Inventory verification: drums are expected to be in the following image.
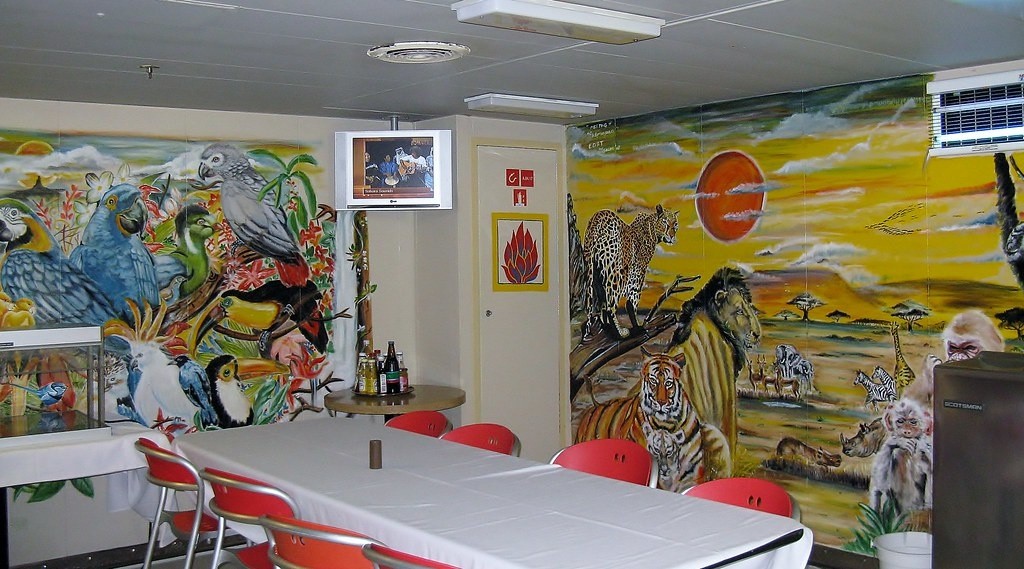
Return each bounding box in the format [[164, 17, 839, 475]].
[[385, 176, 399, 188]]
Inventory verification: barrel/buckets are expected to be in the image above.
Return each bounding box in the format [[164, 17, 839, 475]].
[[873, 531, 932, 569]]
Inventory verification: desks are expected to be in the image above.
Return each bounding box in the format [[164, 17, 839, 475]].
[[323, 384, 466, 423], [1, 409, 166, 569], [931, 351, 1024, 568]]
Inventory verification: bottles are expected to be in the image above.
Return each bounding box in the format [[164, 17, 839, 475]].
[[365, 360, 378, 395], [383, 340, 400, 393], [358, 339, 374, 362], [373, 350, 383, 359], [368, 353, 378, 361], [395, 352, 405, 369], [377, 357, 388, 394], [358, 359, 368, 394]]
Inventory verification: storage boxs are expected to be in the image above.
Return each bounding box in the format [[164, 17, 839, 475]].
[[0, 323, 112, 448]]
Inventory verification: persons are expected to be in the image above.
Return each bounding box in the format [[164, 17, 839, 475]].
[[366, 146, 433, 191]]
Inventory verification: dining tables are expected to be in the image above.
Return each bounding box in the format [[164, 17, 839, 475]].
[[174, 417, 814, 569]]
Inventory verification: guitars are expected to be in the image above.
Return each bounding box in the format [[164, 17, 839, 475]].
[[399, 162, 427, 176]]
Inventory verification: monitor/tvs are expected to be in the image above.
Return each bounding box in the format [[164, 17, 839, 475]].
[[335, 129, 453, 209]]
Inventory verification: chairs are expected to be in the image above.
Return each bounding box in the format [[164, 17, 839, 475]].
[[131, 410, 803, 569]]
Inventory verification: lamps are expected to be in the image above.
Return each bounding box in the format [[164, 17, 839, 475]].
[[450, 0, 666, 46], [463, 93, 600, 119]]
